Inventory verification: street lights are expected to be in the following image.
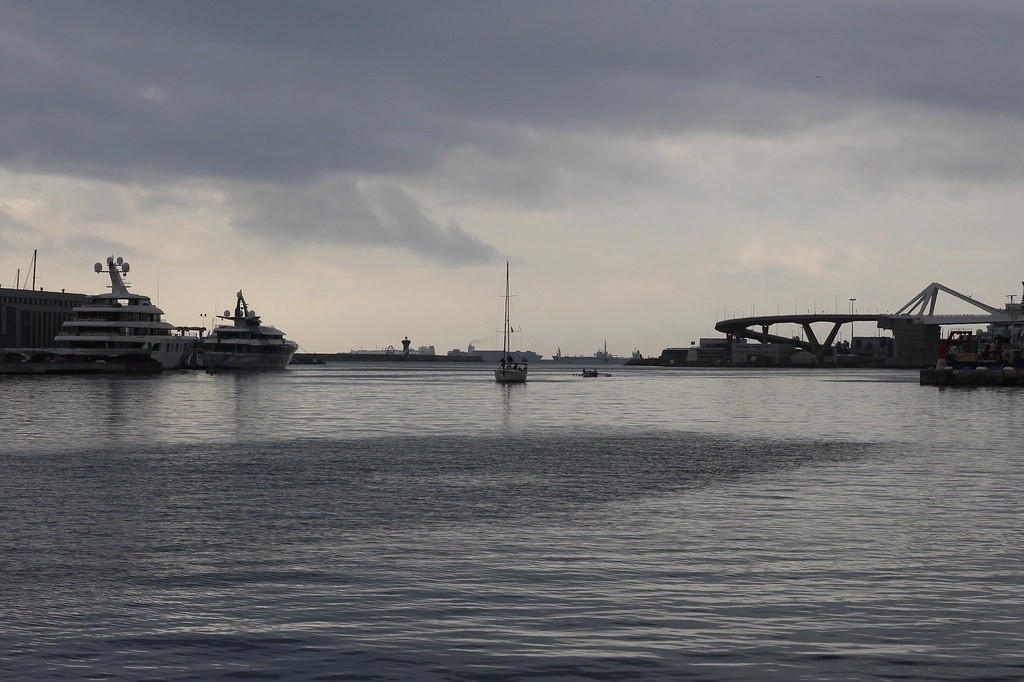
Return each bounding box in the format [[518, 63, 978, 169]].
[[849, 297, 856, 339]]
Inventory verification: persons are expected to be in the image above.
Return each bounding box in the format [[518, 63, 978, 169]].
[[583, 367, 597, 373], [937, 342, 947, 366], [500, 357, 522, 371]]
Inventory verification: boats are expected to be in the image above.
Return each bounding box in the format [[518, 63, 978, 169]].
[[581, 367, 611, 377], [48, 253, 299, 371]]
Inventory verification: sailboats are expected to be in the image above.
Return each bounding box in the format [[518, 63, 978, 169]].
[[494, 259, 527, 383]]
